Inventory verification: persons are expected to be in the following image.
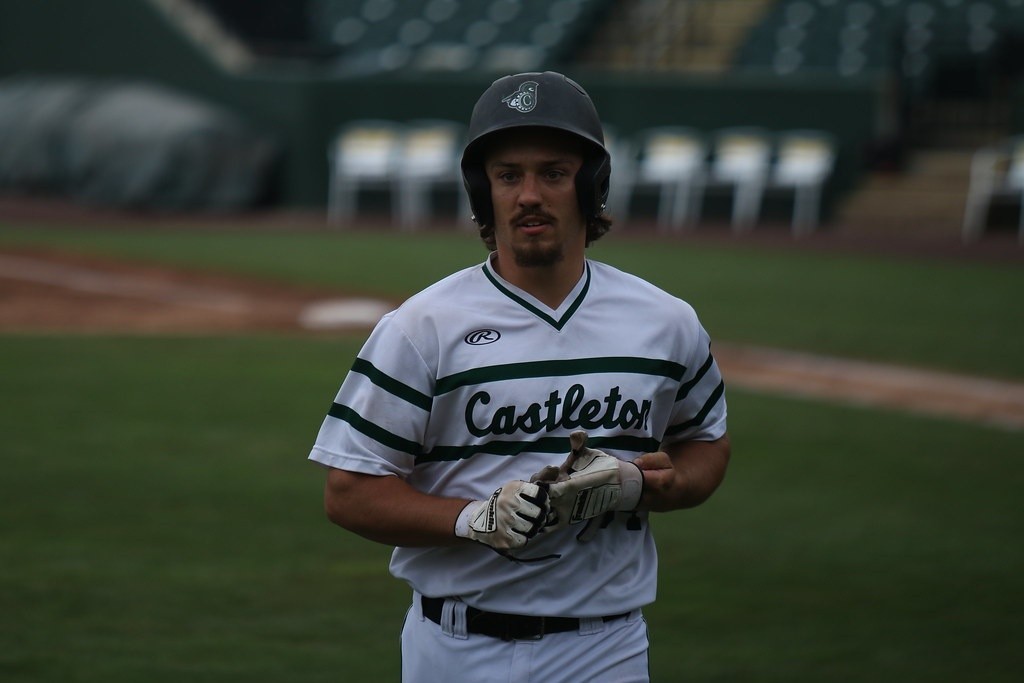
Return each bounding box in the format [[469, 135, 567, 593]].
[[306, 72, 728, 683]]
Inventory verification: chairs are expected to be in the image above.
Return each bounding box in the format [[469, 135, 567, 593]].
[[325, 126, 836, 236]]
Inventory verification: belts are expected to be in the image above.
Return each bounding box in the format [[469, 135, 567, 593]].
[[421, 595, 630, 641]]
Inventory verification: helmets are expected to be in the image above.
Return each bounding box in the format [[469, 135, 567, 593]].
[[460, 71, 610, 226]]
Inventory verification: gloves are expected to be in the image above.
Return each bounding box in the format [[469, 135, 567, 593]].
[[535, 431, 644, 542], [454, 479, 549, 549]]
